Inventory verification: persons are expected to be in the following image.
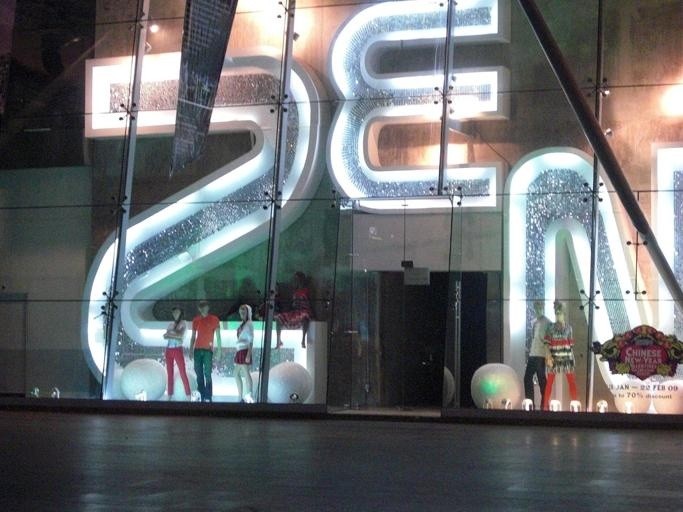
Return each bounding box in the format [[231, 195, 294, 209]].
[[542, 299, 578, 411], [189, 300, 221, 403], [232, 304, 255, 403], [524, 300, 551, 409], [275, 271, 311, 350], [162, 306, 191, 400]]
